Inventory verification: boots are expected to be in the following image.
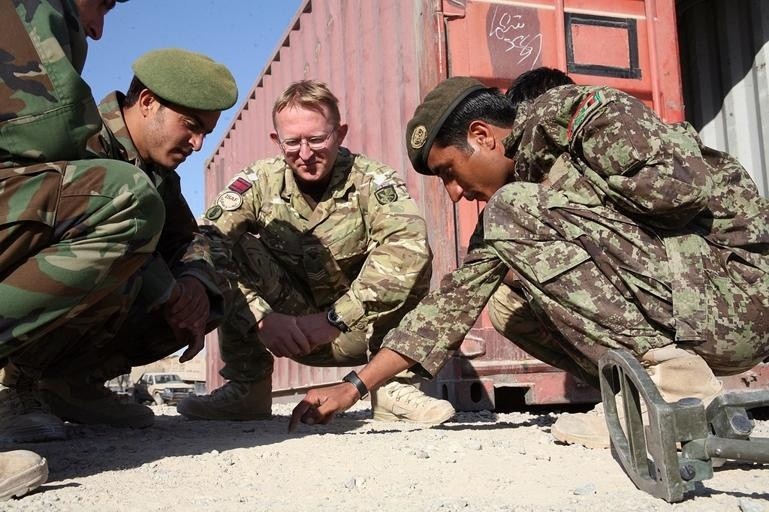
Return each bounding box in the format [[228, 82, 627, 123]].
[[176, 372, 272, 421], [35, 372, 155, 429], [550, 343, 728, 449], [0, 356, 67, 443], [370, 369, 456, 427]]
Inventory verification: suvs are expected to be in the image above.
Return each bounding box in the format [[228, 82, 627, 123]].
[[136, 373, 194, 404]]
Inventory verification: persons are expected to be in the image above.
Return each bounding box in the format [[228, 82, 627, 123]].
[[287, 75, 769, 447], [177, 80, 457, 425], [0, 48, 239, 441], [0, 1, 168, 501], [489, 66, 607, 392]]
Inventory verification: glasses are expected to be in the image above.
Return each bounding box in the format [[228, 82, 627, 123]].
[[277, 119, 339, 153]]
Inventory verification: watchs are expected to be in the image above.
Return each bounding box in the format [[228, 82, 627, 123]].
[[327, 308, 347, 333]]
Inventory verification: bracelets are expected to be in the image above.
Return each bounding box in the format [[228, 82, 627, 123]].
[[343, 371, 368, 399]]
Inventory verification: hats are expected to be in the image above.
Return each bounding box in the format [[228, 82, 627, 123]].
[[406, 77, 486, 175], [131, 48, 238, 111]]
